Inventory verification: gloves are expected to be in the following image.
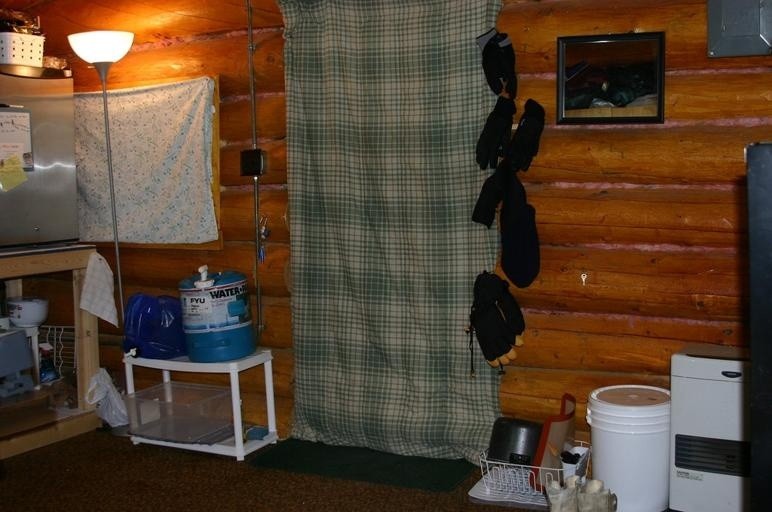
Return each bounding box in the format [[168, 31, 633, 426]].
[[468, 271, 525, 369], [475, 27, 521, 101], [475, 95, 545, 171]]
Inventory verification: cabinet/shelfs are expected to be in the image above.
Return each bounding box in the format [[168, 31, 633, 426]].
[[0, 239, 104, 461], [122, 346, 276, 465]]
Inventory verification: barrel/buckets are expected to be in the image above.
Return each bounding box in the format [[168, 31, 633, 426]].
[[177, 264, 257, 362], [122, 293, 186, 360], [586, 384, 670, 511]]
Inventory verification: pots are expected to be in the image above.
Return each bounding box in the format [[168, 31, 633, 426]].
[[486, 417, 544, 465]]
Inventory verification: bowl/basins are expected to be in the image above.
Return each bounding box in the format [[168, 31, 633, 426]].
[[7, 301, 49, 328]]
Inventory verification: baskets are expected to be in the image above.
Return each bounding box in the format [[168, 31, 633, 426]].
[[0, 32, 46, 67], [478, 438, 591, 495]]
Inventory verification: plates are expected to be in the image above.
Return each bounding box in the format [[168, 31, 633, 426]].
[[1, 64, 44, 78]]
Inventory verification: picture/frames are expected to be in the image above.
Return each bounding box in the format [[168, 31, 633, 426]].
[[554, 31, 665, 127]]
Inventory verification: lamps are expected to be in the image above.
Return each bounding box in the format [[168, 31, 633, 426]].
[[65, 27, 136, 345]]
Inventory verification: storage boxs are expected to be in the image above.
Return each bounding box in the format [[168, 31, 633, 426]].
[[124, 378, 247, 446]]
